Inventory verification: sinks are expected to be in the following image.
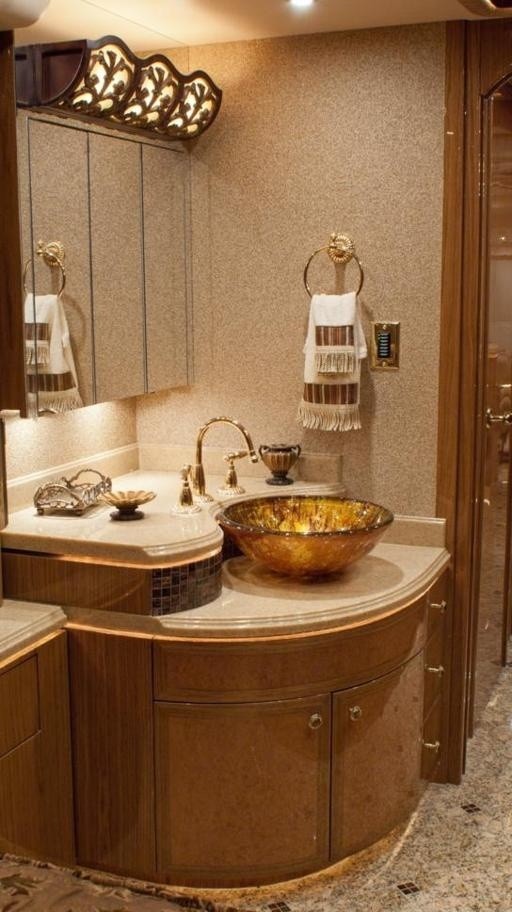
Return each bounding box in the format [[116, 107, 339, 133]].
[[214, 494, 394, 583]]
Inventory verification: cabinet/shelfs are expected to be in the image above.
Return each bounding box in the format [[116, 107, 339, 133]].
[[420, 552, 450, 793], [148, 586, 429, 893], [62, 617, 157, 899], [0, 622, 81, 912]]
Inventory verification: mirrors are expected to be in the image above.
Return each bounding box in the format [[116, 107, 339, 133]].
[[15, 96, 196, 422]]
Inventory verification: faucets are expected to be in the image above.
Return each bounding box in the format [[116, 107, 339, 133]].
[[37, 406, 57, 417], [192, 415, 260, 497]]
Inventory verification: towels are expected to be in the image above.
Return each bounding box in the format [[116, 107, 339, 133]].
[[21, 292, 84, 419], [297, 289, 371, 437]]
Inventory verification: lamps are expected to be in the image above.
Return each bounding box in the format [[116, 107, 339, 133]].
[[14, 32, 224, 142]]
